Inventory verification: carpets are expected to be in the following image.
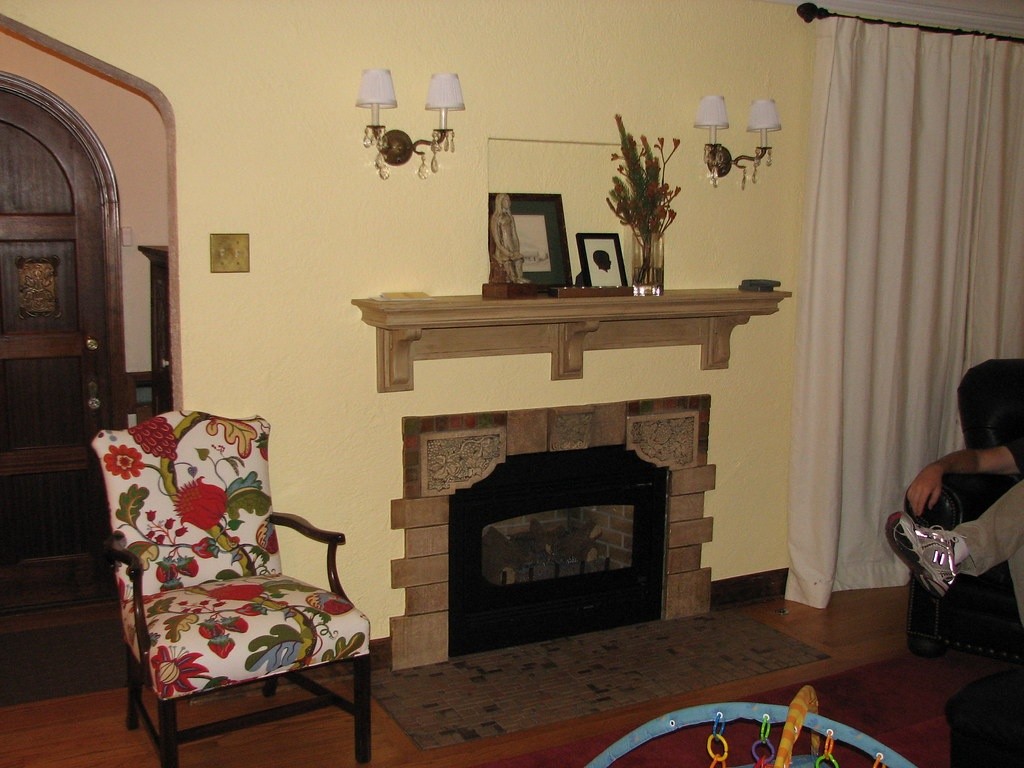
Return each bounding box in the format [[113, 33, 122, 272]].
[[466, 646, 1013, 767], [355, 610, 832, 752]]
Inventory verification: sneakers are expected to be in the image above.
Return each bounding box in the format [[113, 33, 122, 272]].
[[885, 513, 967, 600]]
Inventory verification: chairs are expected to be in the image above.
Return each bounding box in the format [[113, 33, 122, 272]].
[[89, 412, 374, 768], [904, 357, 1024, 666]]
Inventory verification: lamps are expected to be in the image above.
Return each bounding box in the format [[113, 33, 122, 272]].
[[353, 67, 466, 180], [692, 96, 781, 190]]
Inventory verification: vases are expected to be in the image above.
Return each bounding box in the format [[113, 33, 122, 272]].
[[630, 231, 666, 297]]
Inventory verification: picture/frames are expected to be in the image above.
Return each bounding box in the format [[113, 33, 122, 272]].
[[486, 192, 575, 288], [575, 232, 627, 288]]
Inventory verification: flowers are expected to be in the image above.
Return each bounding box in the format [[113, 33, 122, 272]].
[[604, 112, 683, 286]]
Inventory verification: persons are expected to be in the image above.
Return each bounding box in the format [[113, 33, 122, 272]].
[[888, 432, 1024, 628], [491, 194, 530, 283]]
